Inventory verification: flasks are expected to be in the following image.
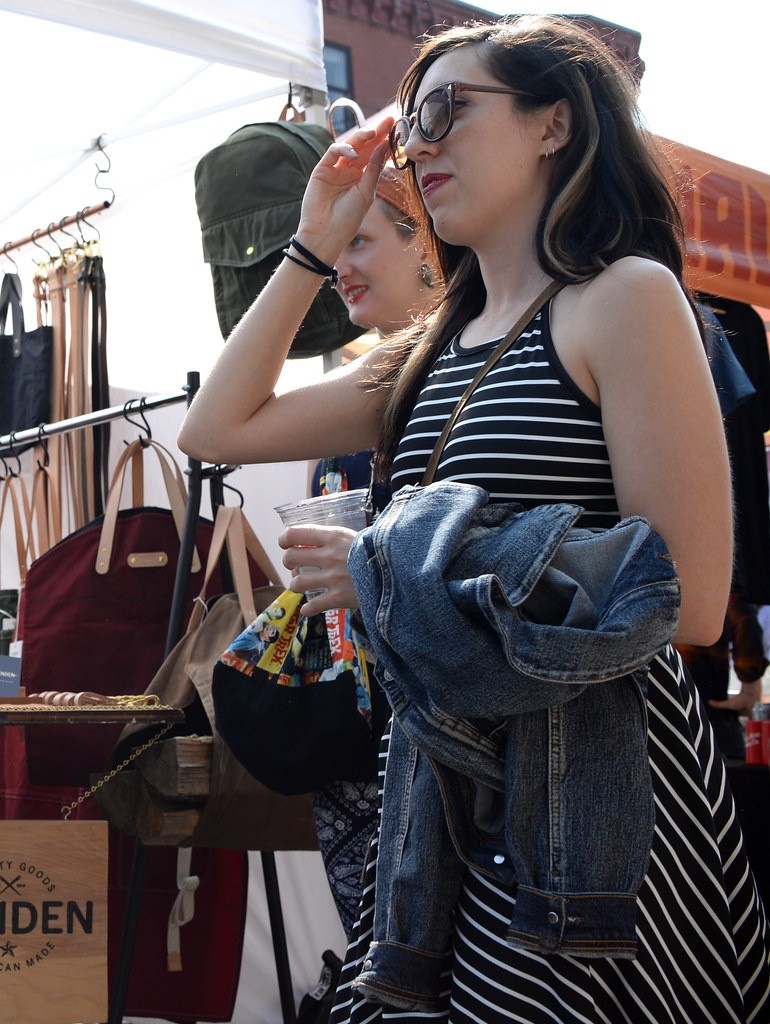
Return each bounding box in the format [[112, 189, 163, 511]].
[[745, 701, 770, 763]]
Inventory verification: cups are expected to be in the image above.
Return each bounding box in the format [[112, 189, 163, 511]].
[[275, 490, 372, 602]]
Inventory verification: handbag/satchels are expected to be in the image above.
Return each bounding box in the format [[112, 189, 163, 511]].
[[88, 506, 320, 853], [19, 436, 268, 790], [212, 571, 392, 794]]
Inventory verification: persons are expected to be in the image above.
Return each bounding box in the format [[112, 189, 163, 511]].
[[679, 586, 770, 768], [229, 605, 286, 651], [308, 157, 448, 940], [176, 14, 770, 1024]]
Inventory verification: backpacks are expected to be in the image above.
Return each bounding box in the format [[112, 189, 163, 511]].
[[194, 102, 373, 360]]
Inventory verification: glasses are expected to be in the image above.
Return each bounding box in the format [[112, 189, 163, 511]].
[[387, 79, 551, 170]]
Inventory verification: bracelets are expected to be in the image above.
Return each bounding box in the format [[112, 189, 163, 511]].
[[277, 235, 339, 291]]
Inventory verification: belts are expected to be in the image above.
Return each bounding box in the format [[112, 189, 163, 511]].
[[0, 237, 113, 644]]
